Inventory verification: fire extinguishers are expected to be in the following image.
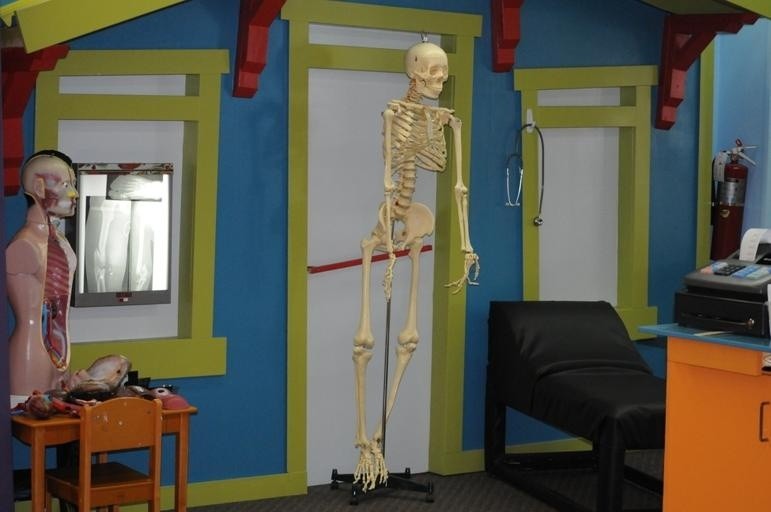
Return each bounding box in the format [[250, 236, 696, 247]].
[[710, 139, 758, 261]]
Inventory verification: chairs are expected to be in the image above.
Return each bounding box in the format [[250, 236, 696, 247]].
[[484, 299, 665, 512], [44, 396, 163, 512]]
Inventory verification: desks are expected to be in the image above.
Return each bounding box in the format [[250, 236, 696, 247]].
[[635, 322, 771, 512], [10, 404, 198, 512]]
